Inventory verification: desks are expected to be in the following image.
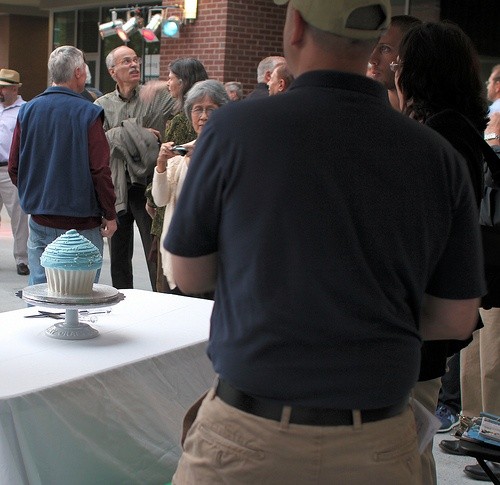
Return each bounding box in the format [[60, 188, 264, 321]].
[[0, 288, 219, 485]]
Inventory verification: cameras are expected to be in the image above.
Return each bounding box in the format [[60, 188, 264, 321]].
[[171, 147, 187, 156]]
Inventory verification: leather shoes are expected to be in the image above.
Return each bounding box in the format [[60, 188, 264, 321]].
[[441, 439, 500, 462], [464, 460, 500, 481]]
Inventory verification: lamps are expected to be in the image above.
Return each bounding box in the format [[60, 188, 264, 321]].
[[114, 9, 145, 43], [160, 7, 185, 38], [137, 9, 164, 43], [97, 11, 127, 40], [183, 0, 199, 23]]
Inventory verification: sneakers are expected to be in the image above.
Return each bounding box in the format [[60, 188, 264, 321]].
[[433, 406, 459, 433]]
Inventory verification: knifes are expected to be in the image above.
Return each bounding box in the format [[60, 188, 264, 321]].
[[25, 310, 111, 323]]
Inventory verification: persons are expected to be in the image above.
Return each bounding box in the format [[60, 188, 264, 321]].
[[162, 0, 488, 485], [0, 68, 30, 275], [141, 16, 500, 483], [93, 45, 177, 294], [8, 45, 118, 308]]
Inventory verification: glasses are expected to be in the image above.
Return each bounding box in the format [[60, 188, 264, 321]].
[[485, 79, 500, 87], [188, 107, 216, 116], [390, 61, 404, 72], [112, 57, 143, 68]]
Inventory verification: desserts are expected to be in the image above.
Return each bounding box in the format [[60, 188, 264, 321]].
[[40, 229, 103, 295]]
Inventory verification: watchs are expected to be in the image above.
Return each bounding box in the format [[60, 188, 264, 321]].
[[484, 133, 497, 141]]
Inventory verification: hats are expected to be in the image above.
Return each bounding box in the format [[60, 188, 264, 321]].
[[272, 0, 392, 40], [0, 69, 22, 87]]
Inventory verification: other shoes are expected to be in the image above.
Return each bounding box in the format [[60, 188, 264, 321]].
[[18, 262, 29, 274]]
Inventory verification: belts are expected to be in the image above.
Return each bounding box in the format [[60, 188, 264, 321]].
[[216, 382, 405, 426]]
